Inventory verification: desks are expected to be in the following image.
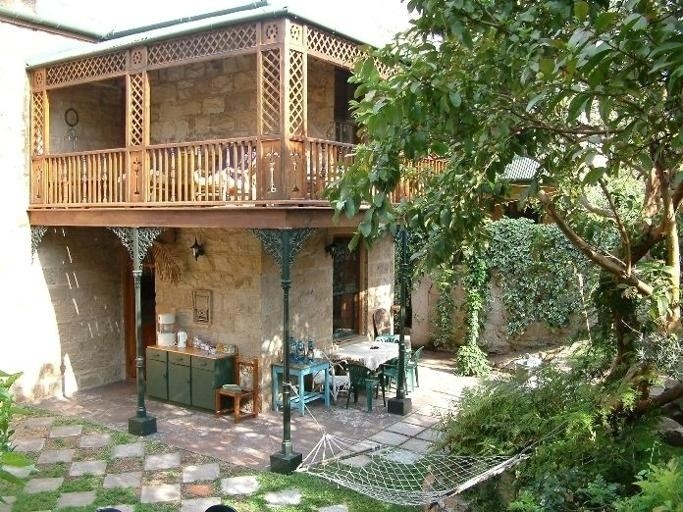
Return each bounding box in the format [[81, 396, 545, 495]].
[[269, 357, 332, 417]]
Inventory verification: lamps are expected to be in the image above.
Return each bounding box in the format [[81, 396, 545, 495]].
[[323, 238, 337, 260], [189, 235, 205, 262]]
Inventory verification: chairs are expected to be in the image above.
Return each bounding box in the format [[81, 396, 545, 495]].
[[213, 355, 260, 426], [310, 335, 425, 413], [187, 167, 257, 201]]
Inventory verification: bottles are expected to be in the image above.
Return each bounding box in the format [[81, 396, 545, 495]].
[[176, 327, 187, 348], [288, 336, 313, 364]]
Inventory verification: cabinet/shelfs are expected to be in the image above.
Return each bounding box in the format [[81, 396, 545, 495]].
[[189, 354, 235, 414], [145, 346, 167, 401], [167, 350, 191, 408]]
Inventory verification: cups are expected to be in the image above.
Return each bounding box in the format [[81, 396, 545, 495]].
[[191, 335, 235, 355]]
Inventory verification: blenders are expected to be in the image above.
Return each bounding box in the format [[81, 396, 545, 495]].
[[155, 313, 175, 348]]
